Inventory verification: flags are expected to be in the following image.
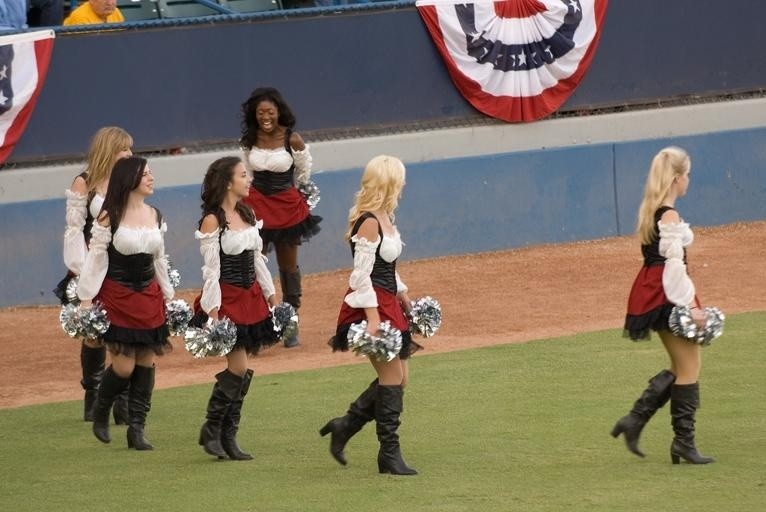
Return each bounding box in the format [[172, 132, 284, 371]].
[[0, 29, 55, 165]]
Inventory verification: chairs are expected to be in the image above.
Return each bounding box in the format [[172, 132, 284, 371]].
[[58, 0, 284, 26]]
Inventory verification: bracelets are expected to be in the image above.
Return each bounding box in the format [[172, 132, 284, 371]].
[[689, 306, 699, 310]]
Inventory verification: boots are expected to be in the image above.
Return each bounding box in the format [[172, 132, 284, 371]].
[[221, 368, 253, 460], [199, 368, 243, 457], [319, 379, 384, 464], [611, 370, 676, 457], [81, 338, 157, 450], [375, 385, 416, 475], [279, 266, 301, 347], [671, 383, 714, 464]]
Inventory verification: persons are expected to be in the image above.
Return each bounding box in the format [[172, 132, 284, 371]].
[[610, 145, 726, 464], [52, 126, 134, 424], [77, 157, 175, 450], [319, 155, 424, 476], [0, 0, 30, 29], [63, 0, 126, 33], [187, 157, 280, 460], [239, 87, 323, 347], [31, 0, 64, 26]]
